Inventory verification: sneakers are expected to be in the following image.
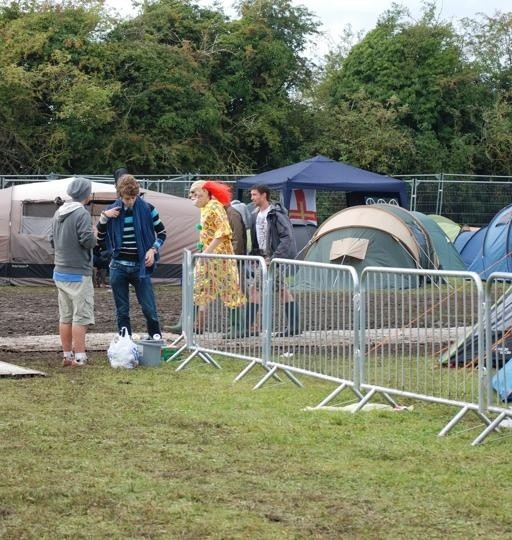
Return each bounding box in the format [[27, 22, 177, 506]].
[[63, 355, 89, 366]]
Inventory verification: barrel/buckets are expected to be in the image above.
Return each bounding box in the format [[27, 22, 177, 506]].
[[132, 339, 165, 368]]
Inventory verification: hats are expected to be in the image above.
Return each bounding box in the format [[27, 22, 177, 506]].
[[67, 178, 91, 201]]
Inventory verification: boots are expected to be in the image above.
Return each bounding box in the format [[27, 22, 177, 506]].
[[163, 305, 198, 333], [222, 302, 262, 338], [276, 302, 302, 337]]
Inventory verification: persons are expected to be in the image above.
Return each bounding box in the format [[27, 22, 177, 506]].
[[96, 173, 167, 340], [45, 178, 98, 368], [163, 179, 301, 334]]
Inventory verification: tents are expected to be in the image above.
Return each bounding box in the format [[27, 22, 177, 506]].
[[294, 202, 468, 291], [426, 204, 512, 282], [288, 220, 317, 253], [0, 178, 202, 287]]
